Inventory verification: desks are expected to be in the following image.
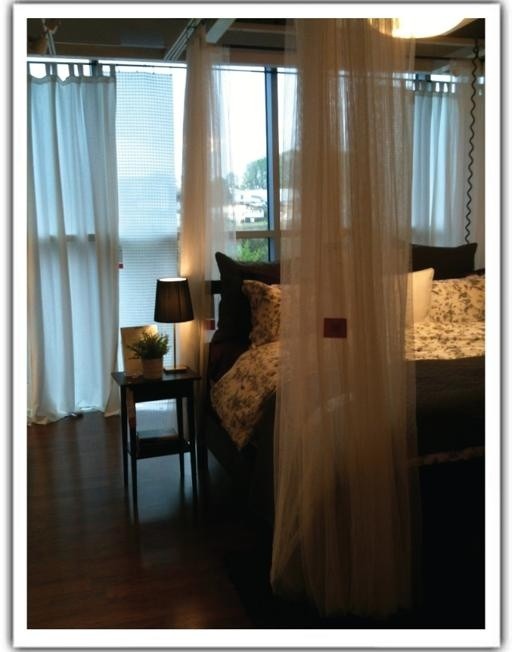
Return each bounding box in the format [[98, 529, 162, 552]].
[[111, 365, 201, 529]]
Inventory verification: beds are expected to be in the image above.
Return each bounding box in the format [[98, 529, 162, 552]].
[[184, 280, 485, 627]]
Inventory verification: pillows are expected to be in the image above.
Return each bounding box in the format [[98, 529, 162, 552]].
[[209, 243, 485, 366]]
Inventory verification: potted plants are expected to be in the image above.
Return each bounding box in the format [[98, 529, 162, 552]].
[[127, 332, 169, 378]]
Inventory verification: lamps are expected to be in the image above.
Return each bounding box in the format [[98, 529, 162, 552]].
[[154, 277, 194, 374]]
